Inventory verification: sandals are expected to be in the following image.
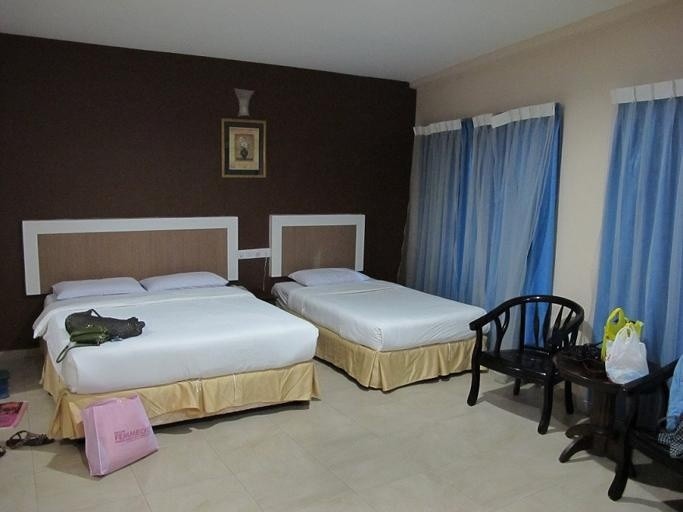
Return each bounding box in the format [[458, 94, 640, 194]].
[[0, 430, 56, 457]]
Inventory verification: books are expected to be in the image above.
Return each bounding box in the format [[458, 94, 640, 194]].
[[0, 401, 28, 429]]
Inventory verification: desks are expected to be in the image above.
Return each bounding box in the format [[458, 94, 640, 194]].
[[550, 346, 663, 479]]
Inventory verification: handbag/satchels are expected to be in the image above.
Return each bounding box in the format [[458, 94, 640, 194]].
[[601, 308, 649, 385], [82, 392, 158, 478]]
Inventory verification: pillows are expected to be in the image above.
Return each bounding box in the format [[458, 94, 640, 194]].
[[50, 270, 231, 302], [286, 266, 370, 287]]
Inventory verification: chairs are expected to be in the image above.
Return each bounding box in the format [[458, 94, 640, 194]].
[[467, 294, 586, 435], [606, 358, 683, 505]]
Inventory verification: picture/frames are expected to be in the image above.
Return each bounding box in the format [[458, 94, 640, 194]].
[[219, 116, 267, 181]]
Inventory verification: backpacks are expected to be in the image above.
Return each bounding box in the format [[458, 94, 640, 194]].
[[65, 309, 146, 347]]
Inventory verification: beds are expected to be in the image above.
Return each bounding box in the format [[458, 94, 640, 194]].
[[17, 213, 316, 443], [267, 210, 490, 389]]
